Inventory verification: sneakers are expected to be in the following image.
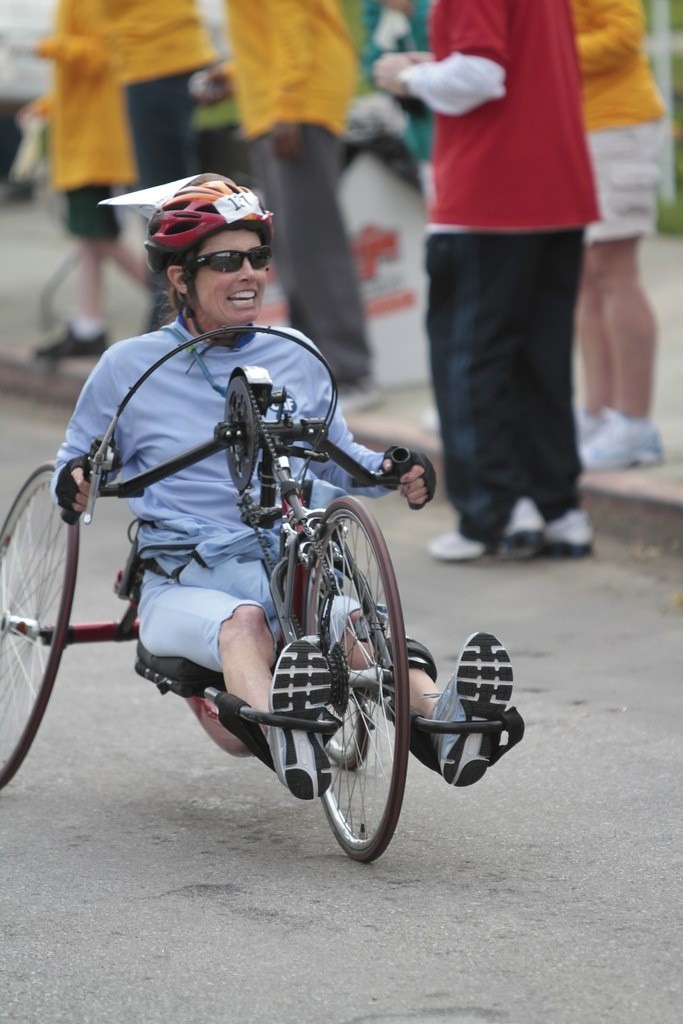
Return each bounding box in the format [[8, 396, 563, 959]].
[[265, 638, 334, 803], [421, 630, 514, 788]]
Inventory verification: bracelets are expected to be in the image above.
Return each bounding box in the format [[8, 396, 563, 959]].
[[398, 67, 410, 94]]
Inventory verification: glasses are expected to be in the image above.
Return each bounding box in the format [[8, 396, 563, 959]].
[[184, 245, 273, 272]]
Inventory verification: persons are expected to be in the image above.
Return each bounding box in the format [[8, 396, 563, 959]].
[[566, 0, 668, 472], [189, 1, 373, 390], [55, 173, 516, 799], [343, 0, 426, 201], [1, 3, 155, 356], [372, 0, 603, 565], [91, 0, 216, 189]]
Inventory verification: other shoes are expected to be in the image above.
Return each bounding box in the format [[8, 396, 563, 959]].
[[578, 406, 665, 470], [496, 508, 594, 553], [34, 321, 108, 357], [427, 531, 487, 561]]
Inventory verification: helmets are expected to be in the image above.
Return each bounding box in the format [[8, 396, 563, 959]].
[[143, 173, 274, 275]]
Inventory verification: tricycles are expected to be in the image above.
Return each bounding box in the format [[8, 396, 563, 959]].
[[0, 319, 526, 866]]
